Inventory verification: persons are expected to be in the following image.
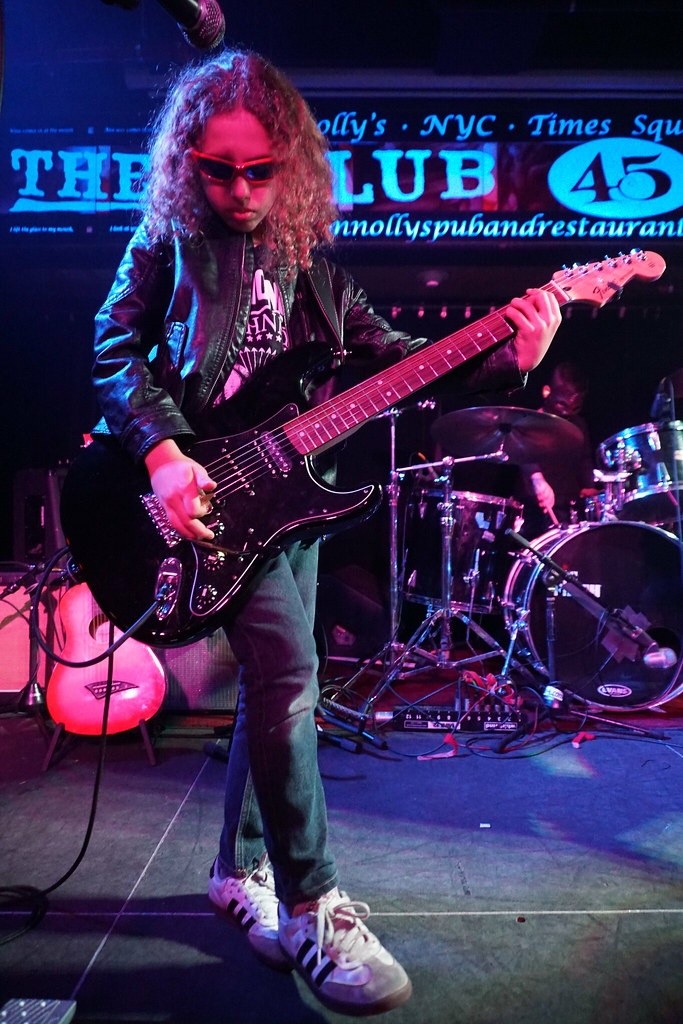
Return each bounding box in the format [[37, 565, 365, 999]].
[[89, 52, 560, 1015], [522, 366, 587, 514]]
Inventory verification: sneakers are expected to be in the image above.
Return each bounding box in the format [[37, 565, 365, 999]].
[[208, 853, 291, 963], [277, 891, 411, 1017]]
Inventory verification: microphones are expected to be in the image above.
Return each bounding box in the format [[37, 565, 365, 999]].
[[155, 0, 225, 54], [630, 626, 678, 668], [650, 377, 667, 419]]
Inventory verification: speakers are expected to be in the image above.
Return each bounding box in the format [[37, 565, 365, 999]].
[[0, 571, 73, 709], [150, 626, 243, 716]]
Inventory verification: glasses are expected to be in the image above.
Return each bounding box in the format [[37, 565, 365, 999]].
[[186, 144, 288, 185]]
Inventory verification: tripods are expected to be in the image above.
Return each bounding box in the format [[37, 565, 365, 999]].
[[323, 400, 665, 754], [0, 556, 59, 757]]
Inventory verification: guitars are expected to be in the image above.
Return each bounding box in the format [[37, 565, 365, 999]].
[[45, 568, 169, 739], [57, 239, 667, 651]]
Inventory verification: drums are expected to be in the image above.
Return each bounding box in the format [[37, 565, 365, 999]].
[[397, 485, 525, 617], [497, 516, 683, 714], [595, 416, 683, 524]]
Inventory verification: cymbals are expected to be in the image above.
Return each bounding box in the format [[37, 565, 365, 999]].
[[428, 403, 588, 472]]
